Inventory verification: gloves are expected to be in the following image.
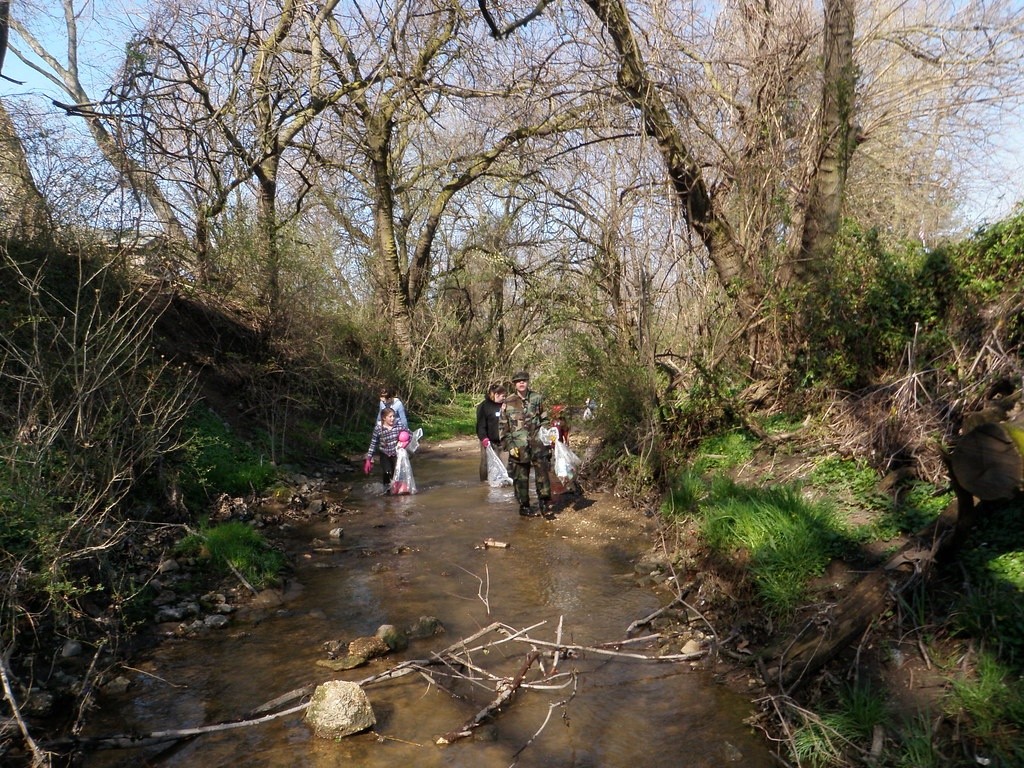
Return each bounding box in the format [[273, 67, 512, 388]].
[[482, 438, 490, 448], [364, 460, 371, 475], [549, 434, 557, 443], [509, 447, 518, 458]]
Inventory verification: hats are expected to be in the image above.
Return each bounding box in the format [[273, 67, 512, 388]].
[[398, 431, 411, 448], [511, 370, 529, 381], [379, 386, 396, 395]]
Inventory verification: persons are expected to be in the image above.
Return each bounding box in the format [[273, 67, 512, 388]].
[[475, 383, 508, 482], [498, 370, 554, 517], [545, 404, 570, 472], [375, 388, 408, 430], [363, 407, 413, 497]]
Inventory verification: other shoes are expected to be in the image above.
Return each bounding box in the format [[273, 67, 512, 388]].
[[519, 503, 540, 517], [539, 500, 554, 519]]
